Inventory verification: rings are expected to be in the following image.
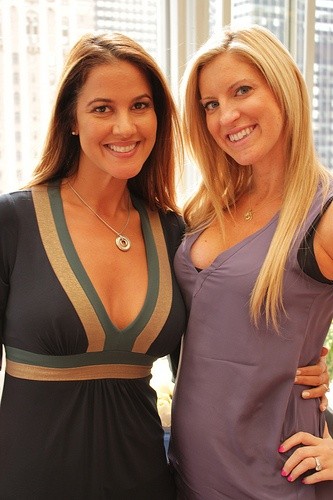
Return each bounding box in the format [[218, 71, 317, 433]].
[[322, 383, 330, 392], [314, 456, 321, 470]]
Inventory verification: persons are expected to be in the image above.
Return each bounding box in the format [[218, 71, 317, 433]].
[[168, 25, 333, 500], [0, 33, 330, 500]]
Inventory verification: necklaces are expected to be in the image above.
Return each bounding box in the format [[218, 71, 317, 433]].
[[66, 179, 131, 250], [244, 186, 283, 222]]
